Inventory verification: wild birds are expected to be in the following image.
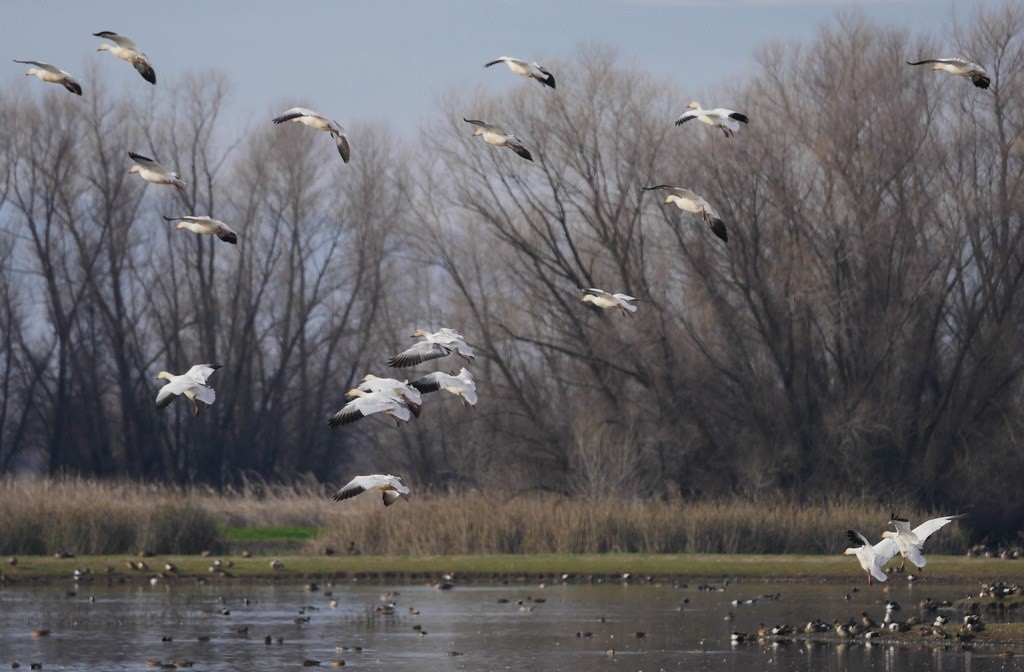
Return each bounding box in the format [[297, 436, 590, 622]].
[[579, 287, 638, 318], [271, 107, 351, 164], [91, 31, 156, 86], [840, 513, 969, 585], [641, 185, 729, 243], [484, 56, 557, 90], [673, 101, 749, 138], [163, 216, 238, 245], [1, 540, 1024, 672], [327, 367, 478, 428], [331, 473, 411, 508], [127, 151, 186, 189], [463, 117, 534, 162], [155, 363, 224, 418], [13, 59, 83, 98], [905, 57, 991, 91], [386, 328, 476, 369]]
